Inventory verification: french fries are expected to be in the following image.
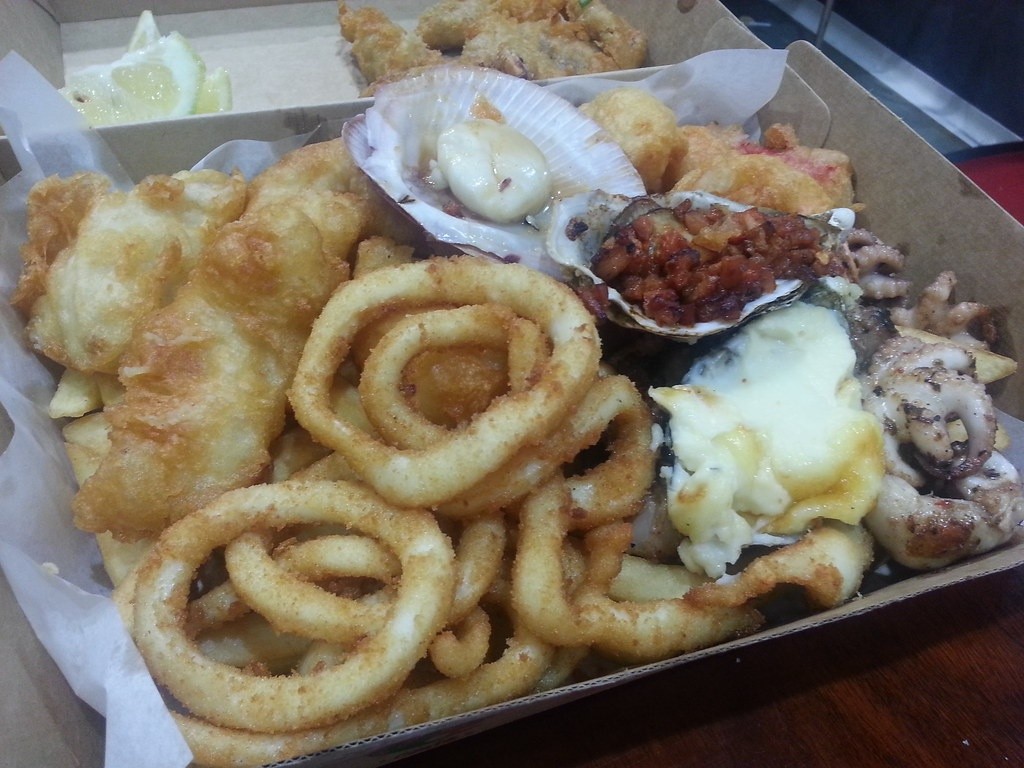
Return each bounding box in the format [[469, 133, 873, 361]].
[[47, 371, 707, 678]]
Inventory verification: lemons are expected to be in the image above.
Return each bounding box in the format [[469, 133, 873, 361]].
[[51, 7, 234, 131]]
